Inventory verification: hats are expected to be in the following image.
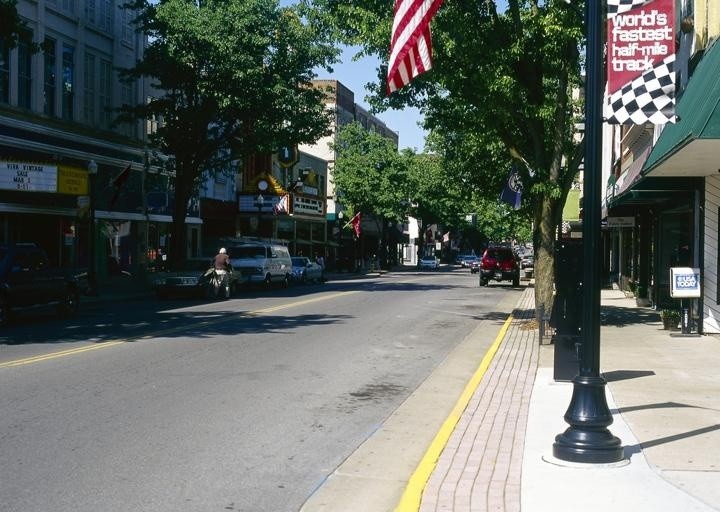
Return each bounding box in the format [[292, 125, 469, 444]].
[[219, 247, 227, 254]]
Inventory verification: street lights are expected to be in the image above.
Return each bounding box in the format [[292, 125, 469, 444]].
[[338, 212, 343, 273], [86, 160, 98, 296]]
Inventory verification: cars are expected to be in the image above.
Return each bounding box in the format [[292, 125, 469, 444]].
[[156, 239, 323, 300], [417, 247, 534, 286], [0, 249, 89, 326]]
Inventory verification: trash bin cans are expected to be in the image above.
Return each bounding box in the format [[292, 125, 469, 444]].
[[525, 268, 534, 278]]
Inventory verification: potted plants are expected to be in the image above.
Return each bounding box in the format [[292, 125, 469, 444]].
[[660, 307, 681, 329], [624, 279, 649, 307]]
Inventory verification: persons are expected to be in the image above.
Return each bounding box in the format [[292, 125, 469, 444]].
[[214, 248, 233, 299]]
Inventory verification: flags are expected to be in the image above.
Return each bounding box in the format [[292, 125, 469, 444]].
[[351, 213, 361, 238], [386, 0, 444, 97], [109, 166, 131, 213], [501, 158, 535, 210]]
